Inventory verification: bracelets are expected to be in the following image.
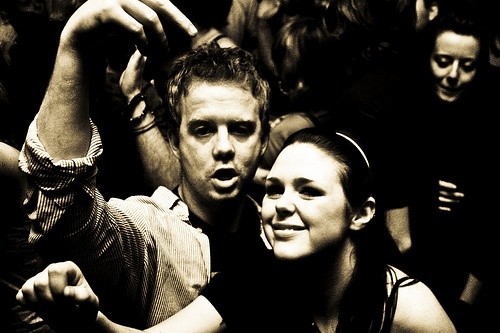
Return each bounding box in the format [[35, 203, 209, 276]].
[[128, 89, 149, 127], [132, 120, 157, 134]]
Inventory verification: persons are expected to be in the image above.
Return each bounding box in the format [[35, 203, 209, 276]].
[[0, 0, 500, 333]]
